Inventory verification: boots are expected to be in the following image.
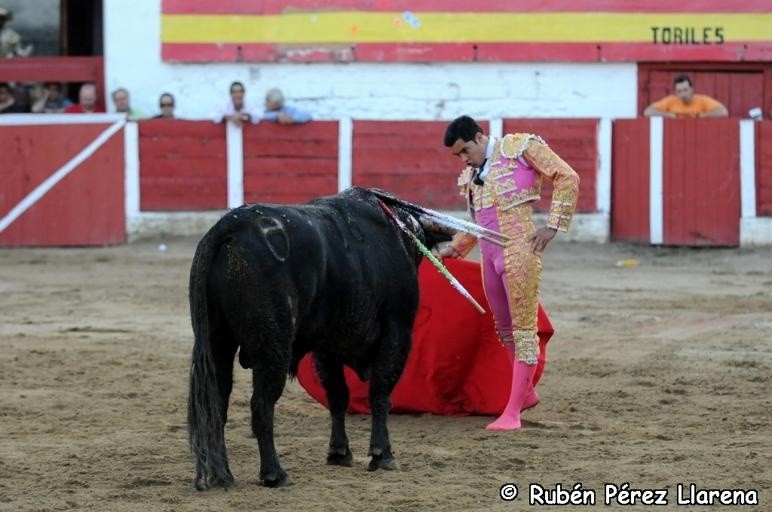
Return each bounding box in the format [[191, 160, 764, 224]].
[[486, 347, 540, 431]]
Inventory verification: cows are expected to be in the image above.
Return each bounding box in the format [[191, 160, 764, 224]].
[[187, 185, 458, 491]]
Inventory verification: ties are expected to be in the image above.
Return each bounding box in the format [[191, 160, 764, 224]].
[[473, 158, 487, 186]]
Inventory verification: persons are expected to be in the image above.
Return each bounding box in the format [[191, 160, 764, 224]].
[[30, 81, 74, 112], [58, 82, 105, 113], [213, 82, 264, 126], [642, 74, 728, 120], [0, 82, 18, 113], [0, 7, 34, 60], [435, 114, 580, 431], [259, 87, 312, 124], [112, 89, 149, 120], [151, 93, 175, 118]]
